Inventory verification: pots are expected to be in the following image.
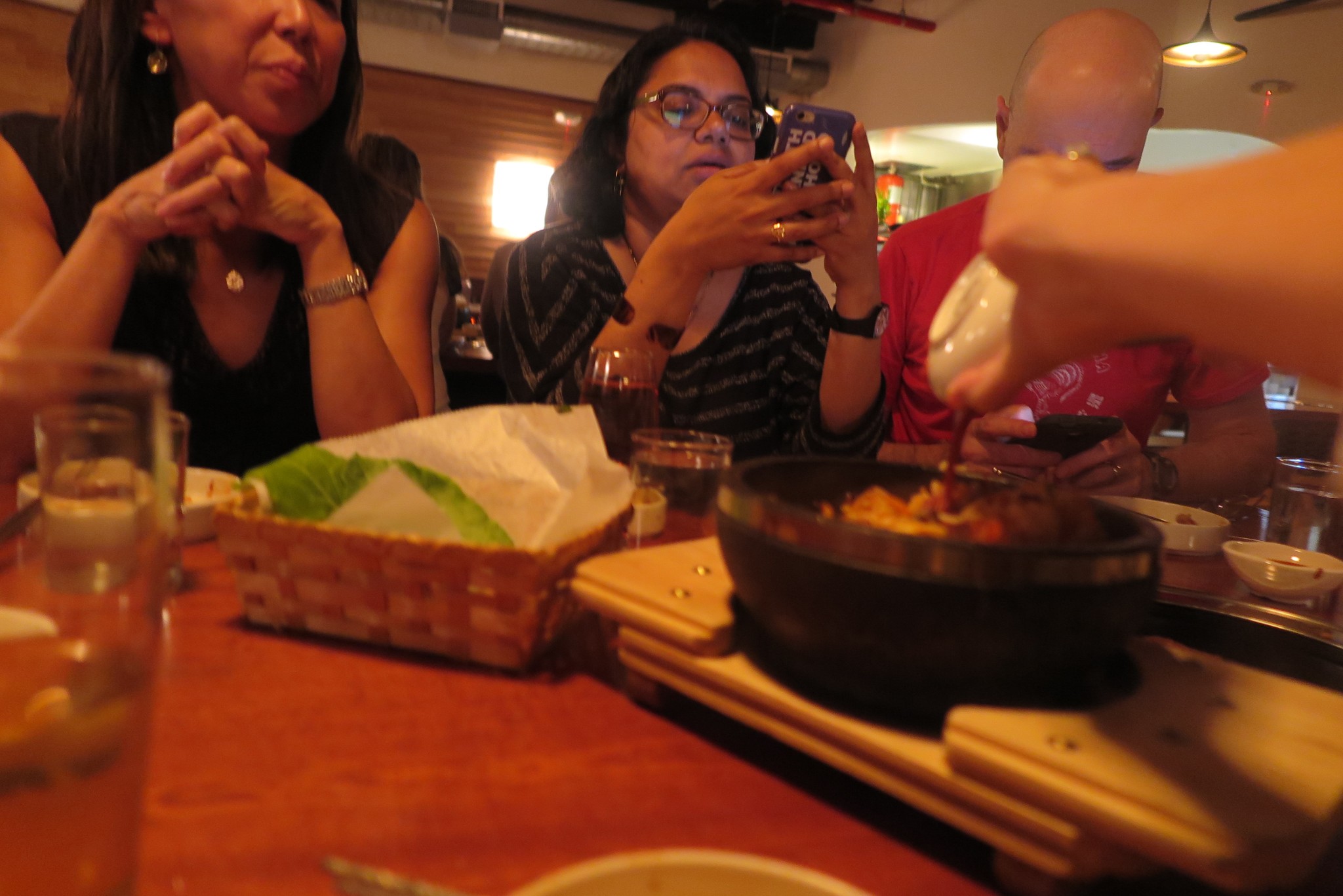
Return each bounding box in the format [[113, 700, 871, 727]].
[[715, 453, 1168, 742]]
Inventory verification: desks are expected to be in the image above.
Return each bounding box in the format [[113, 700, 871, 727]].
[[0, 504, 995, 896]]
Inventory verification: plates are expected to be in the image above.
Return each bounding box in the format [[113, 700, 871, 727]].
[[1086, 495, 1231, 557]]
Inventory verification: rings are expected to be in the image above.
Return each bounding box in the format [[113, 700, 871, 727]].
[[771, 223, 785, 243], [1108, 461, 1124, 479], [993, 465, 1002, 474]]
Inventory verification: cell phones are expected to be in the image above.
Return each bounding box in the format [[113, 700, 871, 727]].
[[770, 102, 855, 264]]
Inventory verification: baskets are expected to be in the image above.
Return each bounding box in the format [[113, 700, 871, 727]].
[[215, 489, 635, 669]]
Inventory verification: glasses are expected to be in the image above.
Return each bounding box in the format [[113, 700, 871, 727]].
[[626, 87, 764, 141]]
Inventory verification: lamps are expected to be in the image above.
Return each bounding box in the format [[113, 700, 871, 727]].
[[1161, 0, 1248, 69]]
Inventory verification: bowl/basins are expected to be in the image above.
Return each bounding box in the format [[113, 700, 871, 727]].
[[16, 456, 242, 544], [626, 483, 668, 535], [1221, 541, 1343, 601]]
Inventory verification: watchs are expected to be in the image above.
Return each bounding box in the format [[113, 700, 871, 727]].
[[300, 264, 370, 307], [1140, 445, 1180, 503], [828, 300, 891, 339]]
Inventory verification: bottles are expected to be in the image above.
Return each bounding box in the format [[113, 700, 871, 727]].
[[874, 164, 944, 234]]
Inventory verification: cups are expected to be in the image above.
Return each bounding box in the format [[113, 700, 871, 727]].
[[1262, 368, 1300, 401], [507, 847, 867, 896], [1265, 455, 1343, 557], [578, 345, 660, 471], [33, 406, 188, 594], [1, 342, 169, 896], [622, 427, 734, 547], [461, 313, 482, 341]]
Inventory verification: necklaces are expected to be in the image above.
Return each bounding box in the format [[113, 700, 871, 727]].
[[620, 231, 713, 331], [217, 238, 250, 292]]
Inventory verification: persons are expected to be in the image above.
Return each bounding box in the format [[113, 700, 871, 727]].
[[1, 0, 567, 480], [946, 127, 1343, 414], [508, 17, 891, 466], [879, 10, 1287, 503]]
